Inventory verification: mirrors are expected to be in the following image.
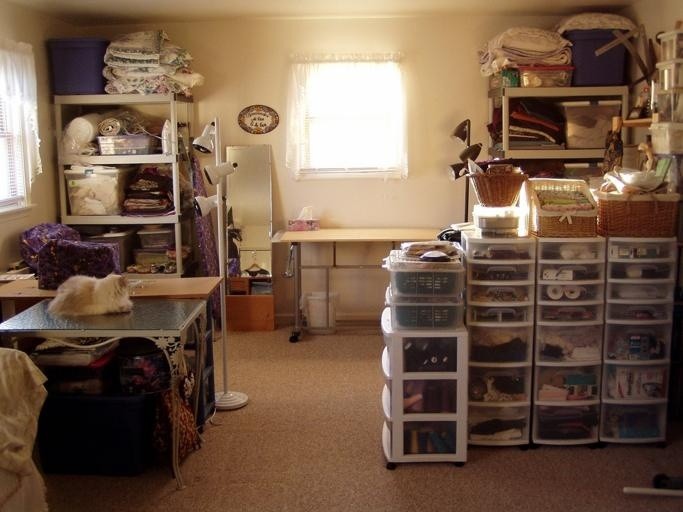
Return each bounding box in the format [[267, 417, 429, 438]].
[[226, 145, 273, 276]]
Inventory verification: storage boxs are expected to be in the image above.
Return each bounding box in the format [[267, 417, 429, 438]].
[[564, 30, 627, 86], [474, 206, 522, 237], [520, 65, 575, 87], [648, 121, 683, 156], [97, 135, 157, 155], [64, 166, 136, 216], [385, 296, 465, 330], [225, 276, 274, 331], [382, 249, 466, 299], [81, 226, 175, 272], [553, 101, 622, 149], [45, 39, 110, 94], [655, 29, 683, 121]]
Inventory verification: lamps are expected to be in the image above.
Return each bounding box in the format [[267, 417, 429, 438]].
[[193, 116, 250, 410], [447, 119, 482, 222]]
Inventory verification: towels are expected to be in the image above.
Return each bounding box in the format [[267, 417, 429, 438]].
[[101, 30, 204, 93], [478, 26, 572, 69]]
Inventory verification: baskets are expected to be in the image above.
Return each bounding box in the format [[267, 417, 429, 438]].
[[597, 192, 681, 239], [394, 272, 455, 295], [396, 306, 455, 325], [466, 172, 528, 208], [529, 176, 599, 238]]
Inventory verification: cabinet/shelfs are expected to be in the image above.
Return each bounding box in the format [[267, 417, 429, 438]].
[[381, 307, 469, 470], [54, 92, 192, 274], [467, 239, 679, 446]]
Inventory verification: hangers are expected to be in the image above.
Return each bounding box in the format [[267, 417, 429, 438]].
[[240, 248, 268, 274]]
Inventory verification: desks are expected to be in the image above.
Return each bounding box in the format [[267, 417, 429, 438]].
[[0, 299, 208, 491], [0, 277, 225, 451], [272, 226, 444, 342]]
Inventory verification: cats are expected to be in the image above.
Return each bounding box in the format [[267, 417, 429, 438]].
[[46, 271, 134, 320]]
